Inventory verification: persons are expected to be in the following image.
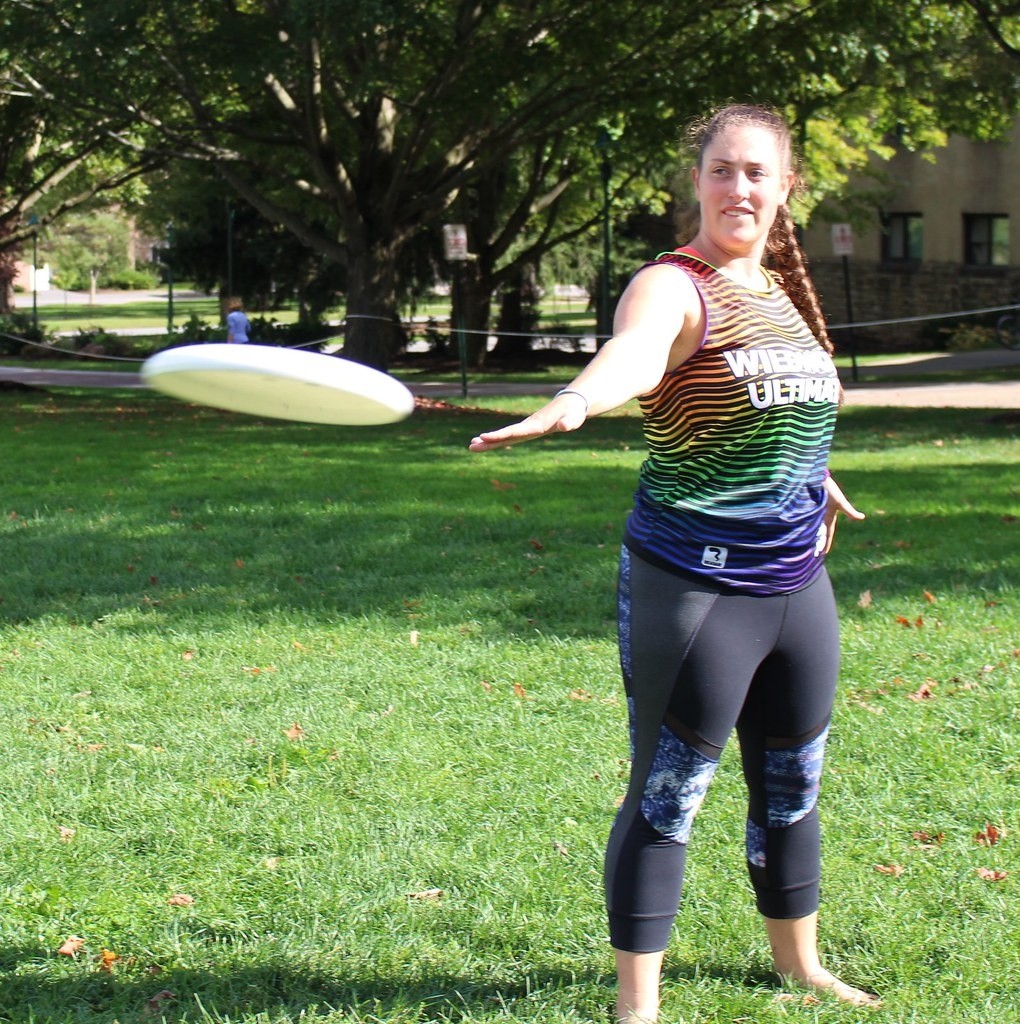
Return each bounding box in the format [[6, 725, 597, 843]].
[[468, 103, 884, 1023], [225, 298, 252, 346]]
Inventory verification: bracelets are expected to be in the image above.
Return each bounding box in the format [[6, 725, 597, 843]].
[[552, 385, 589, 416]]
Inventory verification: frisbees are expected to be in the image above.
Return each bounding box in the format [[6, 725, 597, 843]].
[[130, 344, 417, 429]]
